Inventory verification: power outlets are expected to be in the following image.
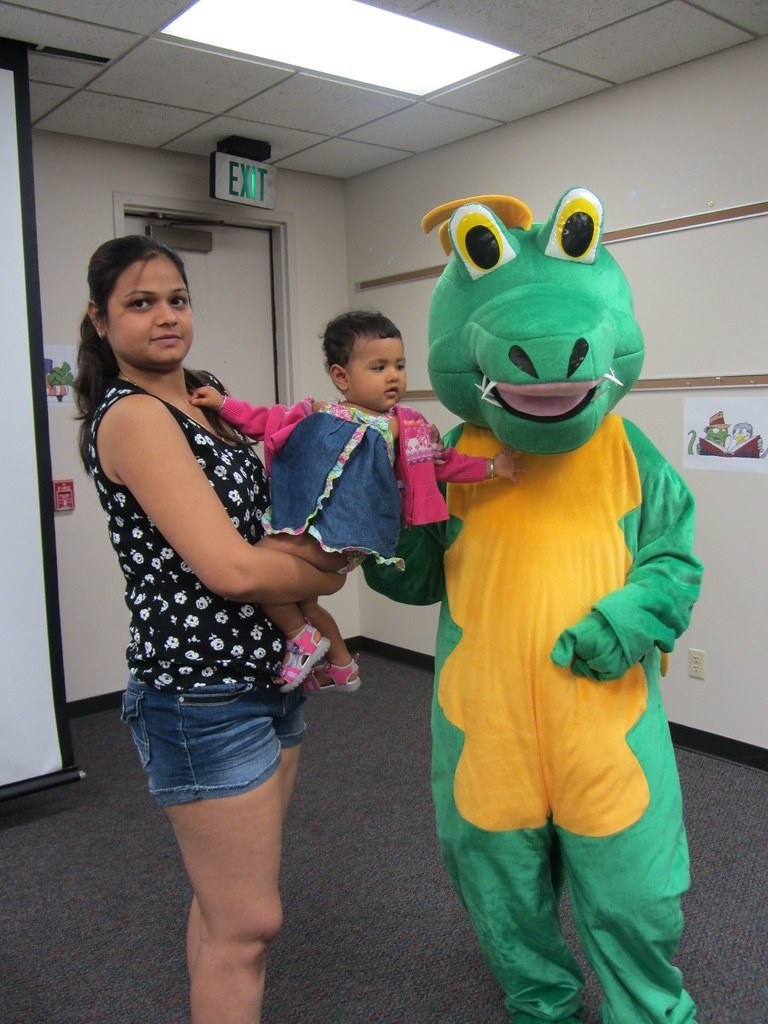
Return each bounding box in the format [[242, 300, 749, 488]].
[[688, 648, 705, 680]]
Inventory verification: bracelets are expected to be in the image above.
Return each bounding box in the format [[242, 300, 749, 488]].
[[219, 394, 227, 409], [490, 458, 495, 481]]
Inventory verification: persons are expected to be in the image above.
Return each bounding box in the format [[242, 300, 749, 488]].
[[68, 235, 346, 1024], [188, 314, 532, 693]]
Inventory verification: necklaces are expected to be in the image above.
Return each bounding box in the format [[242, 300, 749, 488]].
[[119, 372, 226, 443]]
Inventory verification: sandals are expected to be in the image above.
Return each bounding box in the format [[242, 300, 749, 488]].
[[272, 617, 330, 695], [297, 652, 361, 694]]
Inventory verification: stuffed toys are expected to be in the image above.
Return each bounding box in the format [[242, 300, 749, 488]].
[[353, 188, 707, 1024]]
